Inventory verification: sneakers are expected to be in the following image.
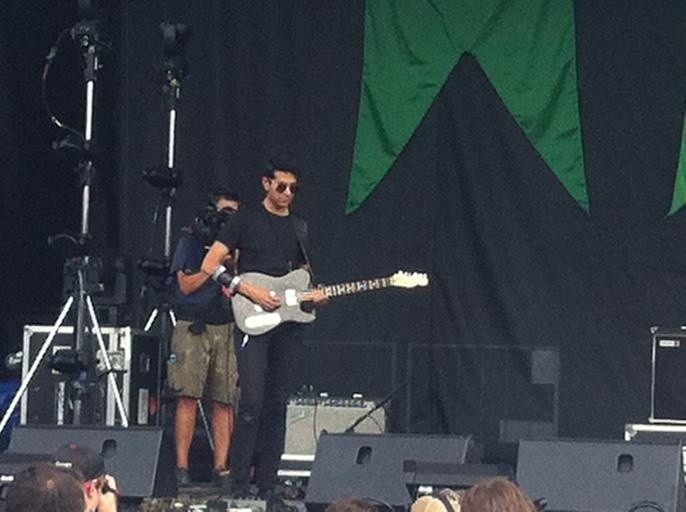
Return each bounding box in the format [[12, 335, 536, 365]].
[[211, 467, 297, 499], [172, 464, 196, 488]]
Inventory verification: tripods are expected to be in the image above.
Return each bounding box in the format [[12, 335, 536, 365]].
[[0, 20, 129, 431], [139, 22, 215, 455]]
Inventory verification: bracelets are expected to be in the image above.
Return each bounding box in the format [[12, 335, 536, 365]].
[[210, 265, 241, 295]]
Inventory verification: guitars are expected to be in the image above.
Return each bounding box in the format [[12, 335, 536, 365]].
[[231, 268, 430, 335]]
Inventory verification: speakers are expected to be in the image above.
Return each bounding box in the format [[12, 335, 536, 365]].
[[515, 438, 686, 512], [304, 433, 482, 512], [0, 426, 178, 497]]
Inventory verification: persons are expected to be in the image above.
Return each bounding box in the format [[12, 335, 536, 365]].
[[202, 156, 331, 500], [50, 442, 119, 512], [4, 463, 86, 511], [325, 477, 536, 512], [164, 188, 241, 488]]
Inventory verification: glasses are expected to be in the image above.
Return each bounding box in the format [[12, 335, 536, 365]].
[[270, 179, 301, 194]]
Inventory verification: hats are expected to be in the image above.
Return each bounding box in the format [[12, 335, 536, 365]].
[[49, 442, 106, 485]]
